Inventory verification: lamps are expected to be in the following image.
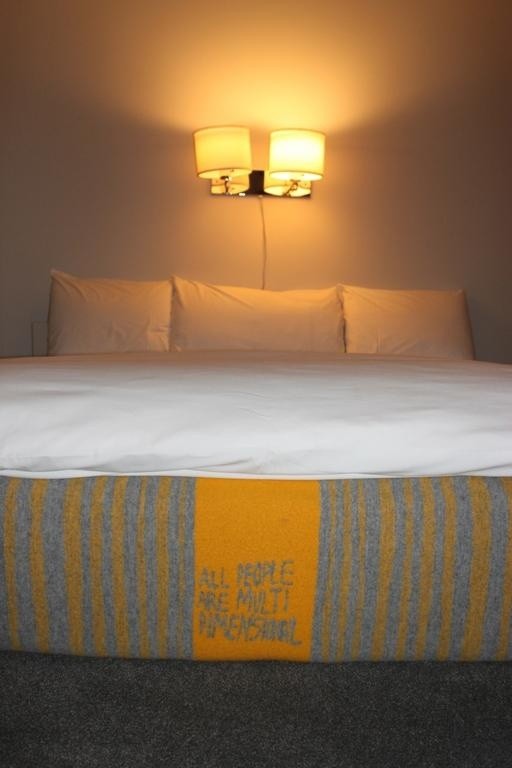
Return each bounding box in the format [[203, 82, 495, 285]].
[[192, 126, 326, 197]]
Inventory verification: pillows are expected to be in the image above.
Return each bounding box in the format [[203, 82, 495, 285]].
[[165, 275, 350, 355], [336, 281, 475, 359], [43, 266, 175, 359]]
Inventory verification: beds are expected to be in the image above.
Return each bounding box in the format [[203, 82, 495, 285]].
[[0, 320, 512, 661]]
